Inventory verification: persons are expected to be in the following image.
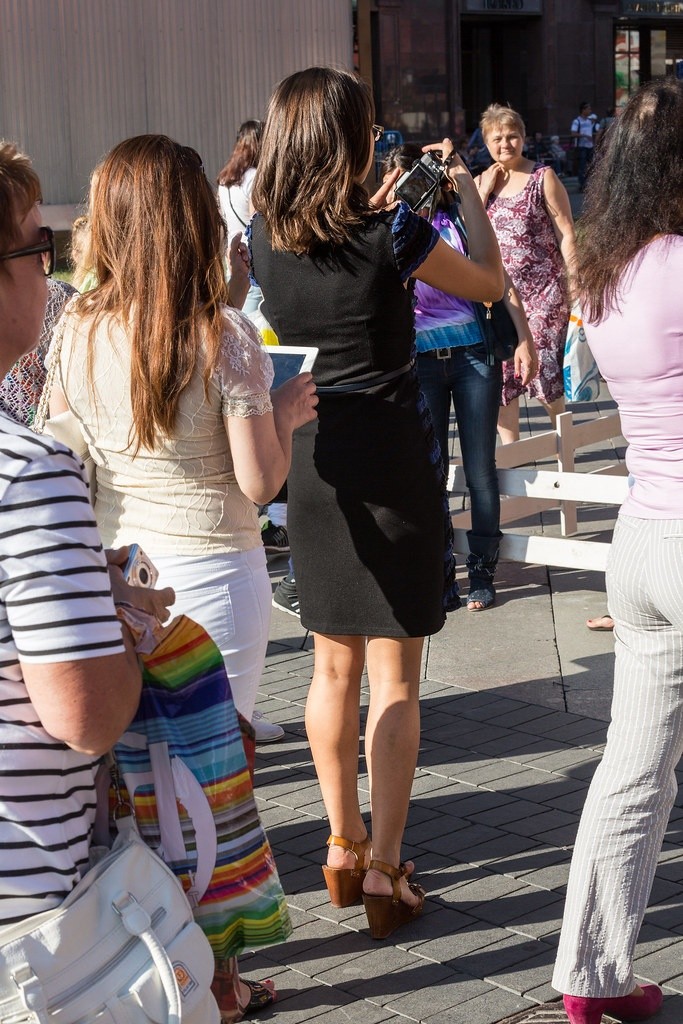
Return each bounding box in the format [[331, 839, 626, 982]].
[[0, 217, 94, 430], [551, 74, 683, 1024], [49, 133, 320, 1024], [571, 103, 616, 191], [522, 131, 568, 177], [474, 107, 578, 445], [213, 121, 502, 617], [251, 68, 507, 936], [0, 141, 174, 930], [466, 124, 489, 173]]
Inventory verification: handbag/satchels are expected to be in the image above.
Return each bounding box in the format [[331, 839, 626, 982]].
[[563, 305, 614, 404], [102, 603, 292, 958], [448, 204, 519, 365], [567, 148, 582, 168], [0, 815, 222, 1024]]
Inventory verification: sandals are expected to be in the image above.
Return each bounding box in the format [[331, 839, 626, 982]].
[[466, 581, 496, 611], [589, 616, 614, 631], [222, 978, 278, 1024], [447, 595, 460, 611]]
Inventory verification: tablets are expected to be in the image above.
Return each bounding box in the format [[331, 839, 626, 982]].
[[260, 346, 319, 391]]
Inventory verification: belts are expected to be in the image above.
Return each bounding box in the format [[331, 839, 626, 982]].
[[416, 341, 484, 360]]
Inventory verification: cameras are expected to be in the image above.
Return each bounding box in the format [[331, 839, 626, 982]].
[[120, 544, 159, 590], [394, 150, 448, 215]]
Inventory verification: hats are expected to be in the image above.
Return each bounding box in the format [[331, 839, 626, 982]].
[[551, 135, 559, 140], [588, 114, 597, 119]]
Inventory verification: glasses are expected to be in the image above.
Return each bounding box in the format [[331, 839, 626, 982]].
[[183, 146, 203, 166], [368, 124, 384, 142], [0, 225, 56, 276]]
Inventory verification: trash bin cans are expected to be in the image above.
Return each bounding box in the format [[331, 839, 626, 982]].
[[374, 131, 403, 184]]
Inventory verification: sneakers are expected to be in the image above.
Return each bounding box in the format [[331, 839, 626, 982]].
[[250, 710, 285, 743], [261, 525, 290, 551], [272, 576, 301, 618]]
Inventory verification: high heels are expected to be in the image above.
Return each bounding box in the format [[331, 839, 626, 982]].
[[362, 860, 425, 938], [563, 984, 664, 1024], [322, 832, 409, 907]]
